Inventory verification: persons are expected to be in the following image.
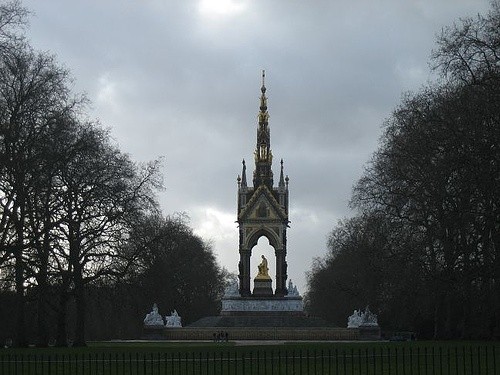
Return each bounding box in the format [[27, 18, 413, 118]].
[[150, 303, 158, 315], [258, 255, 269, 276], [212, 330, 229, 343], [288, 278, 298, 295], [171, 309, 178, 317]]
